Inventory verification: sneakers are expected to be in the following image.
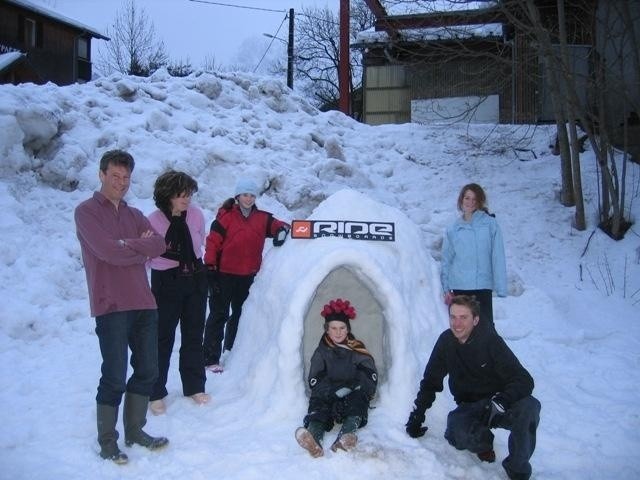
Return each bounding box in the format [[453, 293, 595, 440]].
[[206, 362, 223, 374], [190, 392, 213, 406], [148, 396, 168, 416]]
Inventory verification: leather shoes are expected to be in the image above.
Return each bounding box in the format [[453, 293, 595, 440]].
[[474, 450, 497, 463]]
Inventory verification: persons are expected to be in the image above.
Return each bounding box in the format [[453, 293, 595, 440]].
[[76, 149, 168, 464], [295, 300, 377, 458], [201, 177, 291, 374], [143, 171, 212, 415], [442, 183, 507, 326], [405, 295, 542, 476]]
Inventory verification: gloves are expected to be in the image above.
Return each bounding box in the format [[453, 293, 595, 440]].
[[480, 396, 506, 430], [405, 406, 429, 439], [272, 226, 288, 247], [206, 270, 218, 289]]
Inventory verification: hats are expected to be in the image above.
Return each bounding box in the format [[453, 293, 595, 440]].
[[321, 298, 356, 322], [234, 177, 258, 199]]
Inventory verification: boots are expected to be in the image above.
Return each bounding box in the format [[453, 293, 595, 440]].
[[121, 391, 172, 452], [295, 420, 327, 458], [96, 402, 130, 466], [331, 415, 363, 452]]
